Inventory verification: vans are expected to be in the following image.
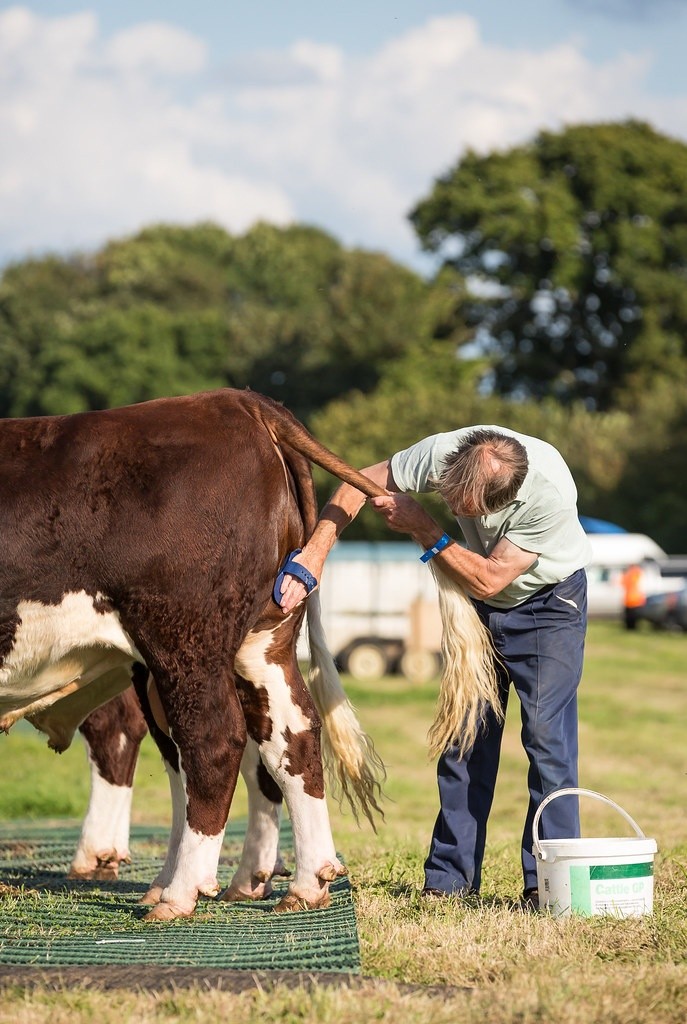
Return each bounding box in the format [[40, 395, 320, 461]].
[[580, 528, 665, 620]]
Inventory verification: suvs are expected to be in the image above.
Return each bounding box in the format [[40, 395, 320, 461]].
[[619, 552, 686, 632]]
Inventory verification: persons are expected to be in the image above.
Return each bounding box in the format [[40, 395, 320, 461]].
[[280, 422, 586, 913]]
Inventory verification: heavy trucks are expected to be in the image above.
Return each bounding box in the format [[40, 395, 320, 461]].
[[291, 535, 446, 685]]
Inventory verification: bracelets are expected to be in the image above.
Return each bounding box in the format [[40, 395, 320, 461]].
[[419, 532, 450, 563]]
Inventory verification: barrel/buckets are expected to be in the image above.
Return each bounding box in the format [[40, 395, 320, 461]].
[[531, 787, 656, 918]]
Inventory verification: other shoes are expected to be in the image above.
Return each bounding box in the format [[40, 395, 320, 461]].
[[413, 888, 482, 915], [521, 890, 539, 915]]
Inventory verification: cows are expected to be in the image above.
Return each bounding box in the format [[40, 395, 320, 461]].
[[1, 388, 511, 929]]
